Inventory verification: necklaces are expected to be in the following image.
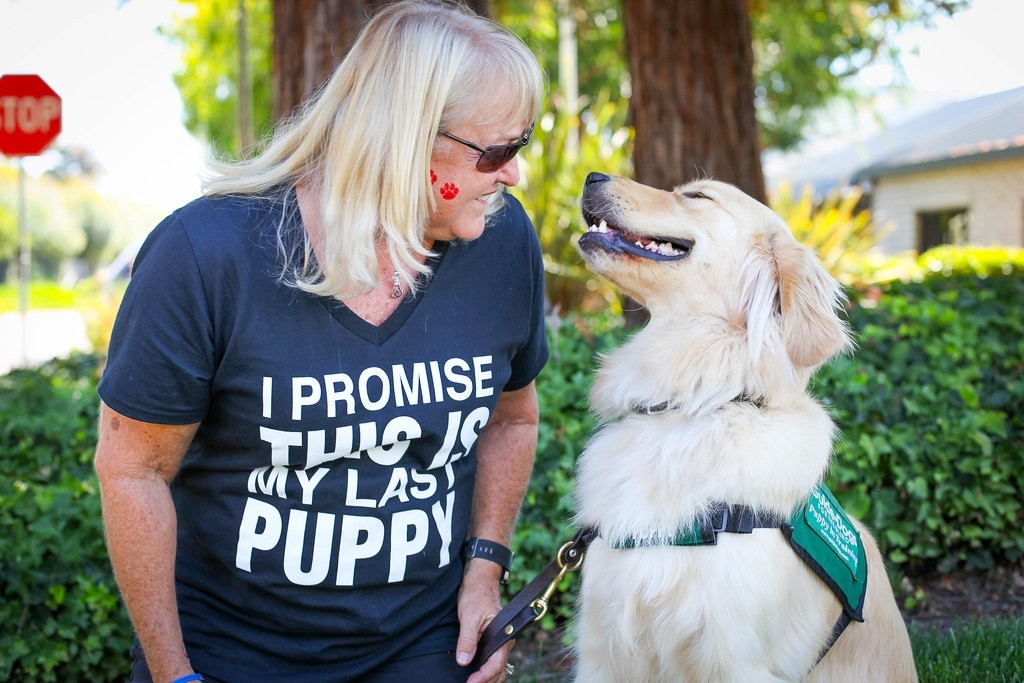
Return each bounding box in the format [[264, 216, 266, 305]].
[[373, 242, 414, 298]]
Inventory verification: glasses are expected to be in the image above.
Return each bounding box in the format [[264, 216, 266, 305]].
[[437, 117, 535, 174]]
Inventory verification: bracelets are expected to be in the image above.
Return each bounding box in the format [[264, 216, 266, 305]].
[[173, 674, 203, 683]]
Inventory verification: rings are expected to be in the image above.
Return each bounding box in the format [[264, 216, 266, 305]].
[[504, 663, 514, 675]]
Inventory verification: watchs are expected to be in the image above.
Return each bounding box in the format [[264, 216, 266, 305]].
[[464, 536, 516, 584]]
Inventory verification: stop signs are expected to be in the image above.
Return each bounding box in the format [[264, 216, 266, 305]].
[[0, 74, 62, 157]]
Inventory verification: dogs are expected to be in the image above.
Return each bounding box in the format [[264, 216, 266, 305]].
[[563, 173, 917, 682]]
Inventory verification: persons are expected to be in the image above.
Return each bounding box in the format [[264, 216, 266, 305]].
[[95, 0, 548, 683]]
